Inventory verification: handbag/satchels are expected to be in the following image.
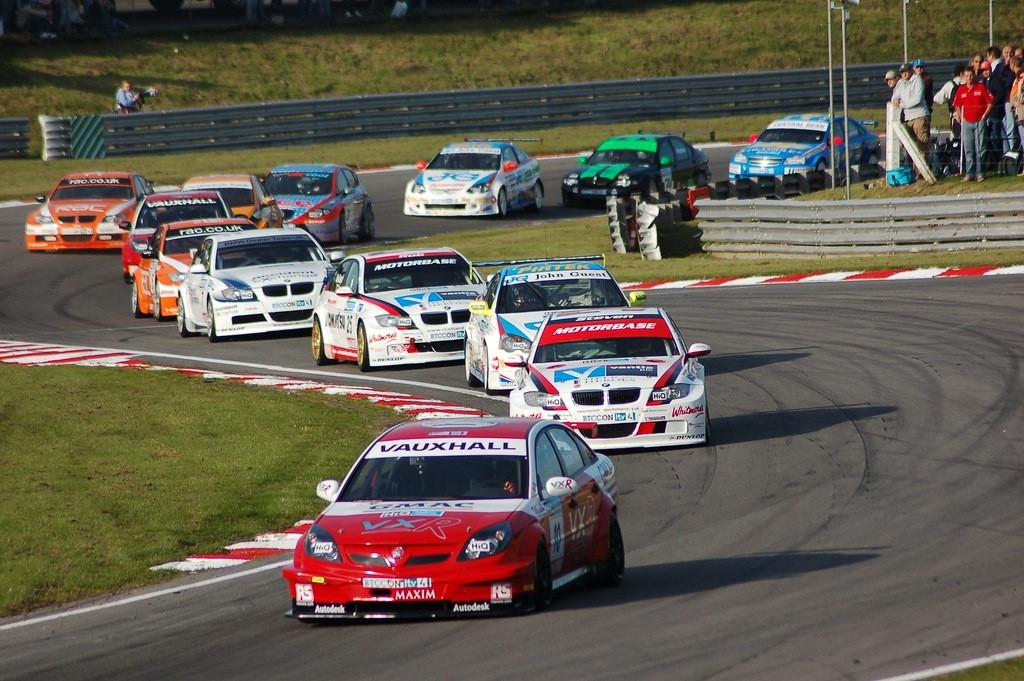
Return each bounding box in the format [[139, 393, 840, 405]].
[[997, 149, 1022, 178], [886, 166, 915, 187]]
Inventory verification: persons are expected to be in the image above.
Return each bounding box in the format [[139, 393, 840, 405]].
[[493, 459, 518, 494], [623, 338, 658, 356], [887, 44, 1024, 183], [116, 81, 158, 115], [257, 0, 364, 25], [511, 284, 568, 307], [0, 0, 131, 40]]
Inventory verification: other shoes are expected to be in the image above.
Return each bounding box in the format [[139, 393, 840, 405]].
[[960, 176, 973, 182], [977, 175, 984, 183]]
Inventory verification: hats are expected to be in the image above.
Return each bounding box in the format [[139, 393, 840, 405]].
[[898, 64, 912, 73], [884, 70, 898, 82], [980, 61, 990, 70], [913, 57, 926, 67]]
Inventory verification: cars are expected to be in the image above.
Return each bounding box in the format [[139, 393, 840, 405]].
[[728, 114, 881, 181], [464, 253, 647, 396], [311, 246, 496, 373], [264, 163, 375, 245], [24, 172, 157, 253], [131, 218, 260, 323], [180, 174, 285, 229], [402, 136, 544, 217], [122, 190, 234, 285], [282, 414, 625, 624], [176, 227, 345, 344], [561, 128, 712, 210], [504, 307, 712, 451]]
